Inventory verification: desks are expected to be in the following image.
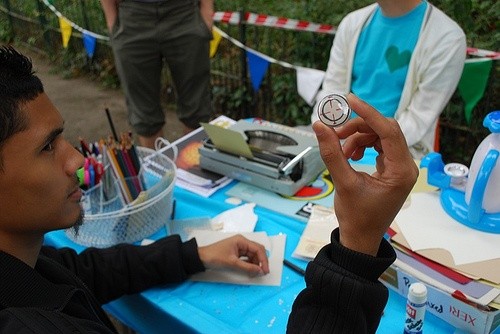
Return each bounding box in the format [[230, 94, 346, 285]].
[[42, 118, 500, 334]]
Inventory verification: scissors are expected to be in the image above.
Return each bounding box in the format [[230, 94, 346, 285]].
[[78, 158, 104, 190]]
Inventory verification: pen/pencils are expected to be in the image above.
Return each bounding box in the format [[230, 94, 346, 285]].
[[73, 137, 102, 165], [102, 130, 144, 202]]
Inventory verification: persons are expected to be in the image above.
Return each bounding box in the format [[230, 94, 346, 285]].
[[0, 44, 419, 334], [311, 0, 466, 167], [100, 0, 216, 151]]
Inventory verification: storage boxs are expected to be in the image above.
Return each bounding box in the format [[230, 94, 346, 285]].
[[396, 267, 500, 334]]
